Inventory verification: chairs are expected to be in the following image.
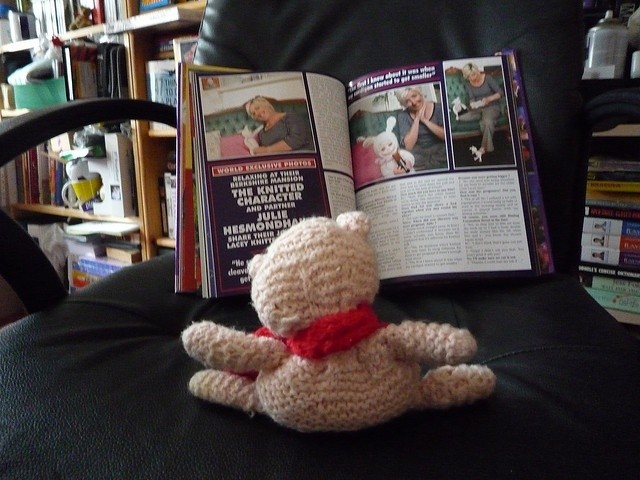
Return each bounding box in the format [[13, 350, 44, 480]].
[[0, 0, 640, 479]]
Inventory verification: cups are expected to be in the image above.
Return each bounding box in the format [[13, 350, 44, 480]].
[[62, 176, 99, 211]]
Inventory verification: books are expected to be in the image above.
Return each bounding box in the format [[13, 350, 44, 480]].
[[577, 124, 640, 323], [28, 223, 142, 295], [0, 133, 141, 219], [61, 40, 129, 101], [175, 48, 555, 298], [144, 34, 200, 131], [157, 150, 176, 240], [16, 0, 128, 36], [139, 0, 171, 13]]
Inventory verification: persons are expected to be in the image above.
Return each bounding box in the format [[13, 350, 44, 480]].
[[242, 97, 312, 156], [462, 63, 504, 164], [394, 84, 448, 171]]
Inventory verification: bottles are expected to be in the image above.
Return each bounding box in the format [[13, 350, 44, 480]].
[[583, 10, 629, 80]]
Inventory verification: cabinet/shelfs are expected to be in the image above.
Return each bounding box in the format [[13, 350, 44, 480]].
[[0, 0, 207, 296]]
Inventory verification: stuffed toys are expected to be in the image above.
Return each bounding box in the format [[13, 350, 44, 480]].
[[363, 116, 413, 174], [184, 210, 499, 433]]
